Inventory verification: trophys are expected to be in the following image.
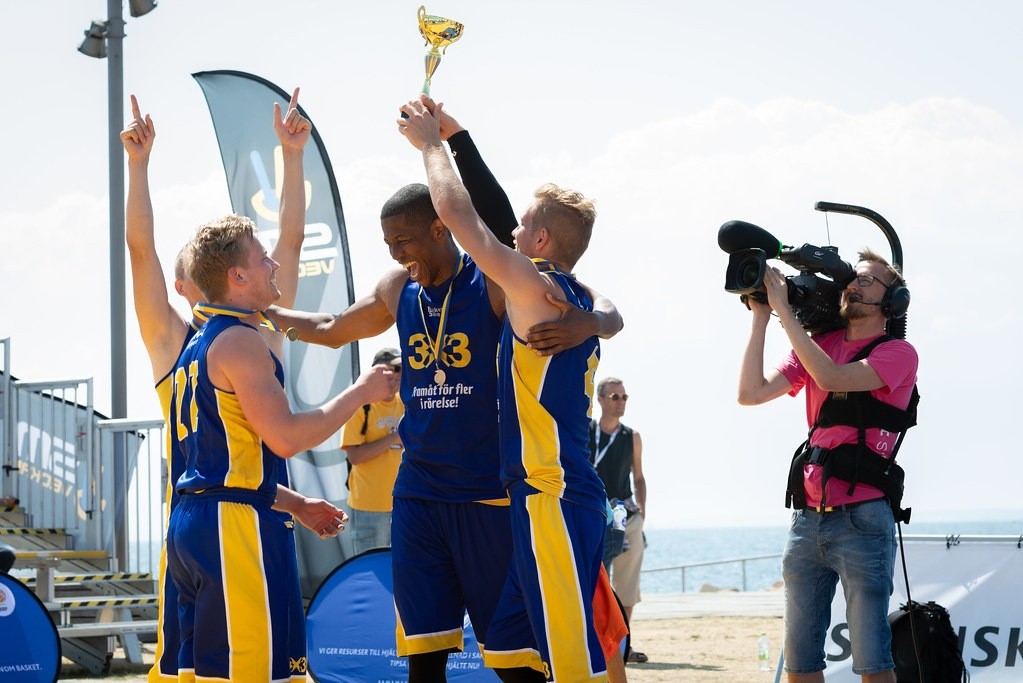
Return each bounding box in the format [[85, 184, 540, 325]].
[[400, 6, 464, 119]]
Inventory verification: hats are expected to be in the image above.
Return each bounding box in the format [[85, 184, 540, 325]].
[[372, 347, 402, 365]]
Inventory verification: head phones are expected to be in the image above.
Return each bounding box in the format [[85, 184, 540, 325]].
[[880, 272, 910, 318]]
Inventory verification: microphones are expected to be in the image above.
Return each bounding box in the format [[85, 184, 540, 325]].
[[849, 295, 884, 306], [718, 221, 784, 260]]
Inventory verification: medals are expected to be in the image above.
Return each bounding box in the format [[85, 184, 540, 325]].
[[434, 369, 446, 386], [286, 327, 300, 342]]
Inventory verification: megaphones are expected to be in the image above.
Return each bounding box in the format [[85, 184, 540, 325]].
[[128, 0, 157, 18], [77, 20, 108, 59]]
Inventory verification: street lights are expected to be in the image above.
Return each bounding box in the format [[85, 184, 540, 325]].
[[78, 0, 160, 578]]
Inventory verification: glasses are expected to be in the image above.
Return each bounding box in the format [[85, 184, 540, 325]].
[[856, 275, 889, 289], [389, 366, 402, 373], [602, 393, 628, 401]]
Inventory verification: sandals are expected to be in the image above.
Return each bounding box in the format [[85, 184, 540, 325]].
[[626, 646, 649, 662]]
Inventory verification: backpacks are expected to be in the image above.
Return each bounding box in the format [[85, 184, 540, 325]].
[[888, 600, 970, 683]]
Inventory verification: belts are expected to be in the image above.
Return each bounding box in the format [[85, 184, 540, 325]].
[[805, 495, 888, 512]]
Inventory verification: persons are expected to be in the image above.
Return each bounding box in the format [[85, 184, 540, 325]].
[[261, 180, 624, 683], [340, 346, 406, 556], [168, 213, 400, 682], [738, 250, 917, 683], [395, 98, 632, 683], [119, 88, 309, 682], [589, 379, 648, 664]]
